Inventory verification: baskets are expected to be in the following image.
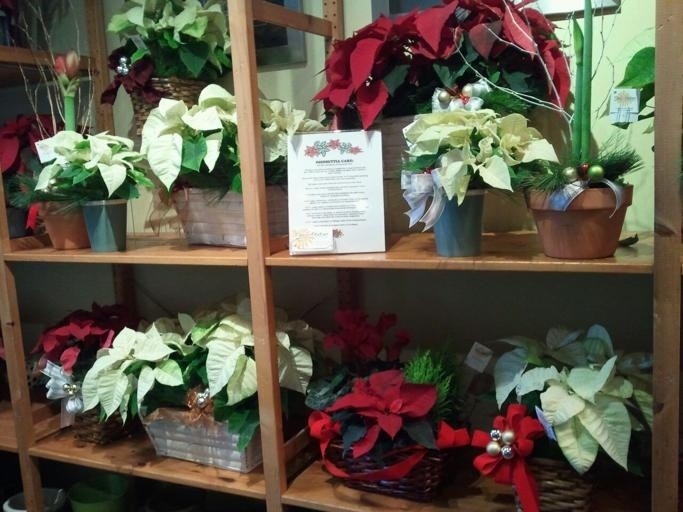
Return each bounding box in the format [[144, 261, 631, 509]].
[[72, 407, 132, 445], [125, 78, 208, 137]]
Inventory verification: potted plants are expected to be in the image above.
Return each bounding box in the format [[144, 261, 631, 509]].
[[5, 149, 91, 250], [505, 130, 645, 259]]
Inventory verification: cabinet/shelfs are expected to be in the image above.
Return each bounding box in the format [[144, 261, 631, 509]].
[[0, 0, 683, 512]]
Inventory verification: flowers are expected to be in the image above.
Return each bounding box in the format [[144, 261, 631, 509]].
[[399, 82, 560, 206], [34, 130, 159, 201], [0, 114, 90, 184], [305, 307, 654, 474], [103, 0, 233, 81], [29, 300, 149, 381], [309, 0, 570, 133], [83, 292, 344, 451], [139, 84, 327, 195]]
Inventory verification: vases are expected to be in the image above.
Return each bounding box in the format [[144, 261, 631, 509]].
[[142, 412, 307, 475], [74, 409, 141, 448], [122, 76, 216, 153], [3, 488, 66, 512], [169, 184, 289, 251], [78, 198, 127, 252], [424, 189, 488, 257]]
